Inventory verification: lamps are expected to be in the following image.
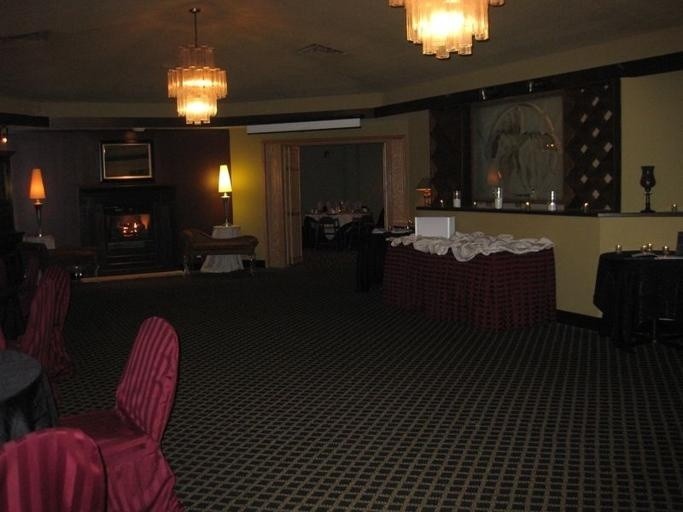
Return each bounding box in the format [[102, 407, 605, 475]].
[[25, 167, 51, 239], [157, 7, 229, 130], [415, 176, 434, 209], [637, 164, 657, 215], [215, 163, 235, 228]]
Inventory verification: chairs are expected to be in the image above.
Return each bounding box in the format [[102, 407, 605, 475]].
[[299, 207, 375, 252], [1, 425, 107, 511], [0, 255, 73, 411], [53, 316, 189, 511]]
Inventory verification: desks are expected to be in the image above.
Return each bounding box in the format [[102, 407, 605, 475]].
[[200, 224, 245, 276], [593, 246, 682, 352], [371, 232, 559, 332], [20, 233, 56, 253], [383, 2, 507, 62], [0, 344, 59, 440]]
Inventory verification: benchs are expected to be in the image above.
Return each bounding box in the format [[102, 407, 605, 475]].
[[18, 240, 99, 279], [173, 229, 261, 280]]
